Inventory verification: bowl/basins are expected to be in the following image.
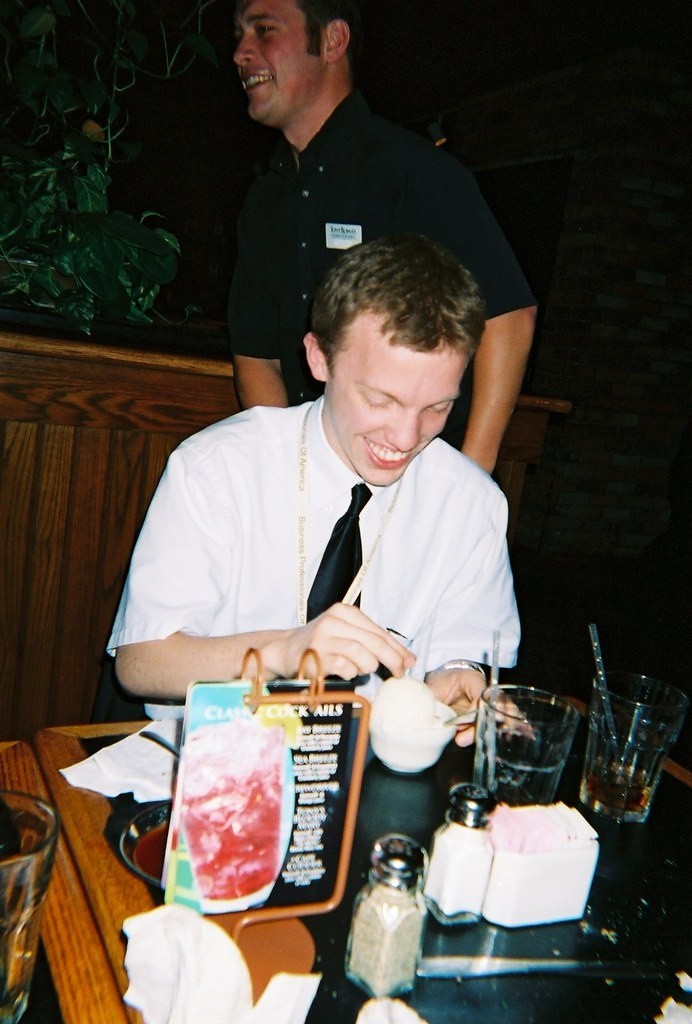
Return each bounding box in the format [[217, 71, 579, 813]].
[[119, 797, 169, 900]]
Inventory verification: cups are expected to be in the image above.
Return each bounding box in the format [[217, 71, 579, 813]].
[[469, 683, 580, 811], [578, 668, 690, 823], [0, 783, 59, 1023]]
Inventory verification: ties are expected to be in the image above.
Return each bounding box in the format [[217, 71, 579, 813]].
[[306, 485, 374, 624]]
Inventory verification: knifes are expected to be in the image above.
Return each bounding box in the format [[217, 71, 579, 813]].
[[416, 956, 684, 981]]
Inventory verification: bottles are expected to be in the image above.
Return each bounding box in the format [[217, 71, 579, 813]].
[[419, 781, 498, 928], [339, 831, 431, 1000]]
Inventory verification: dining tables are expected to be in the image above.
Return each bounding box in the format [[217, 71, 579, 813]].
[[2, 682, 692, 1024]]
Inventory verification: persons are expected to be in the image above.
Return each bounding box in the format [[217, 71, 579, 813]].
[[202, 1, 540, 484], [88, 228, 536, 746]]
